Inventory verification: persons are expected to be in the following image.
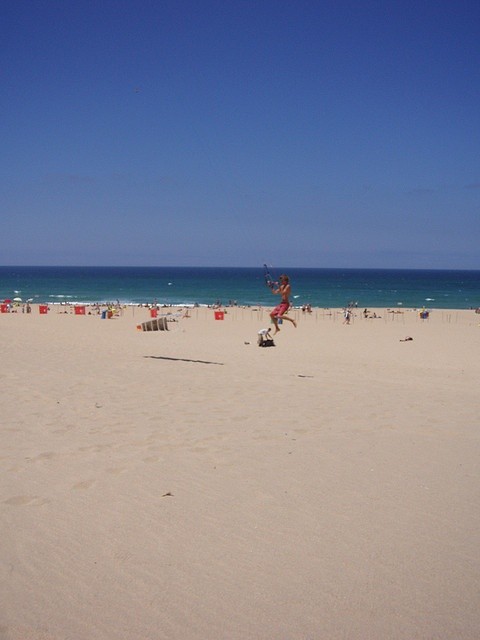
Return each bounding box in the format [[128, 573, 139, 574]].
[[269, 274, 298, 335], [254, 326, 274, 347], [343, 305, 353, 325]]
